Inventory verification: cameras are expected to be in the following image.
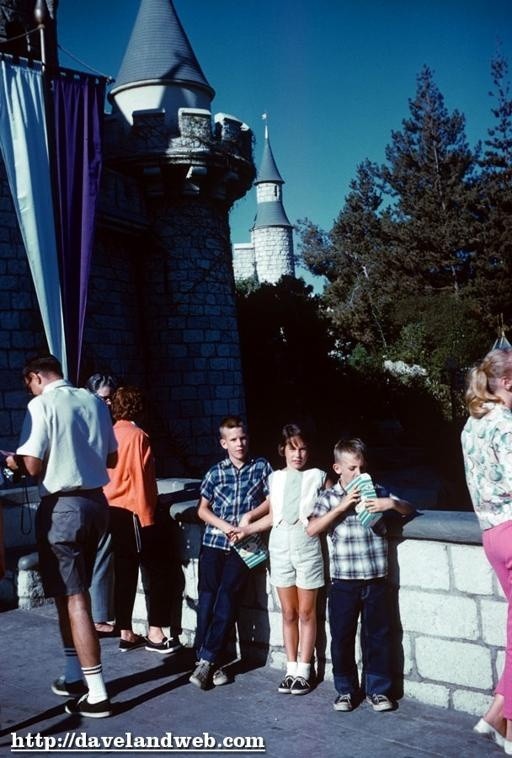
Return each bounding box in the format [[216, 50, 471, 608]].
[[3, 467, 25, 484]]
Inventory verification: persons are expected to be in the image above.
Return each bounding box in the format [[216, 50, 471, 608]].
[[226, 424, 332, 694], [82, 371, 122, 636], [461, 345, 512, 758], [301, 439, 414, 715], [97, 381, 183, 653], [1, 353, 120, 718], [186, 417, 271, 687]]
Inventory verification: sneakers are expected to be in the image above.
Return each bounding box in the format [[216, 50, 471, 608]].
[[188, 656, 229, 689], [50, 678, 89, 697], [117, 634, 146, 653], [333, 692, 359, 713], [63, 689, 114, 718], [364, 690, 395, 712], [144, 633, 182, 655], [277, 674, 316, 695]]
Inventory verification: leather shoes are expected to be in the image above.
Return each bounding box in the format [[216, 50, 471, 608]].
[[473, 718, 512, 755], [95, 625, 121, 639]]
